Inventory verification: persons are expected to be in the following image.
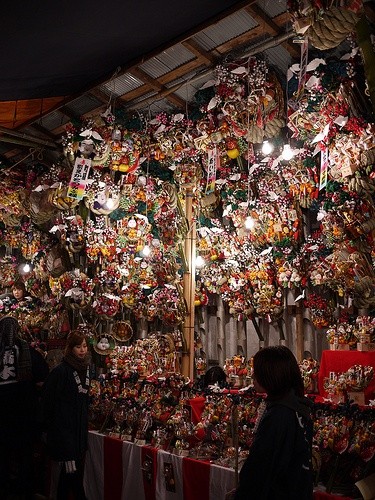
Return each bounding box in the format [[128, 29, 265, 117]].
[[226, 345, 313, 500], [0, 281, 92, 500], [304, 351, 312, 361]]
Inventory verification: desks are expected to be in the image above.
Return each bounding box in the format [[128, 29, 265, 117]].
[[47, 427, 355, 500]]
